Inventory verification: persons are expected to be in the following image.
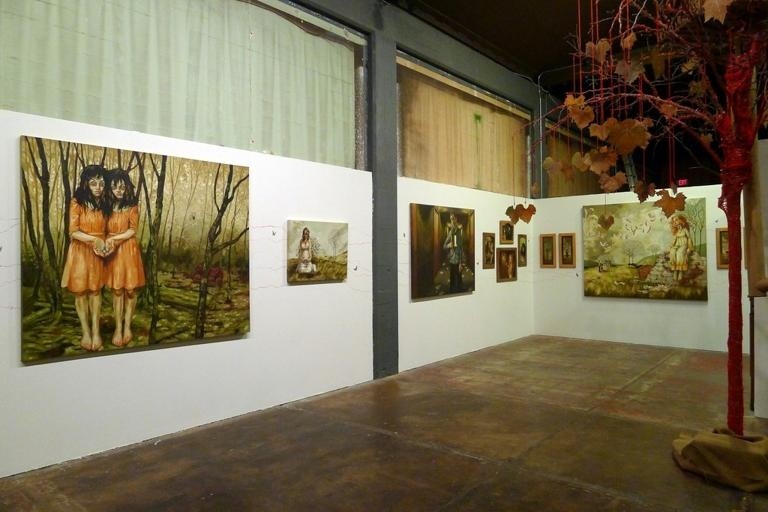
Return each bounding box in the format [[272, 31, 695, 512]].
[[444, 214, 466, 294], [107, 168, 145, 348], [668, 216, 695, 282], [61, 165, 107, 351], [296, 228, 316, 277]]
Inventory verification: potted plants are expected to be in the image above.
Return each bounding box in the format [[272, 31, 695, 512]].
[[496, 248, 518, 283], [517, 234, 527, 267], [483, 232, 495, 269], [716, 229, 730, 270], [499, 221, 514, 244], [559, 233, 576, 268], [539, 233, 556, 268]]
